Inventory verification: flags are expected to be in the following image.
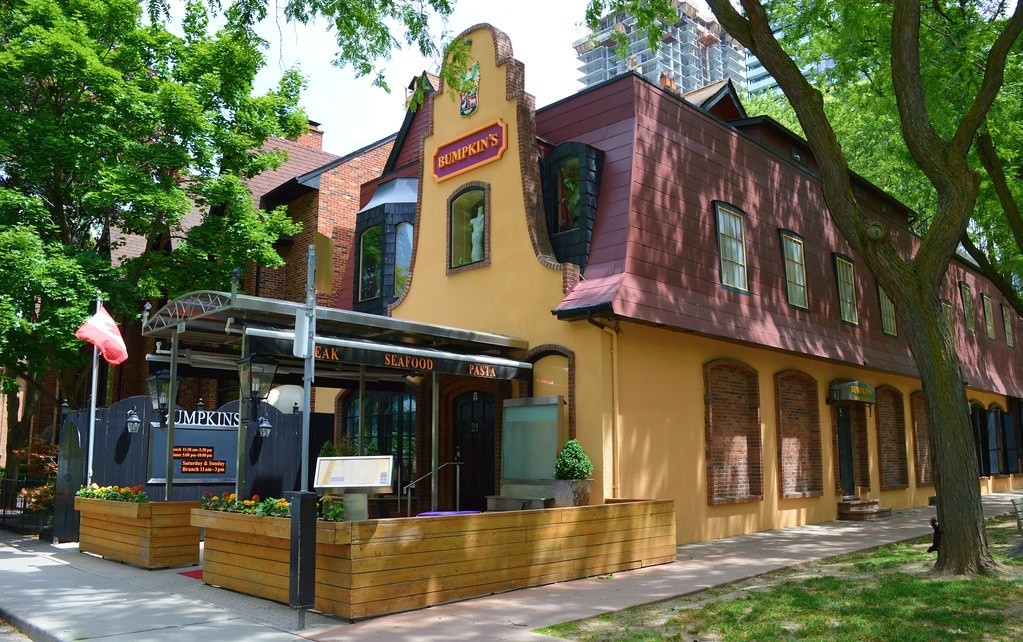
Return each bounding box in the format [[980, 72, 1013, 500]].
[[75, 306, 128, 368]]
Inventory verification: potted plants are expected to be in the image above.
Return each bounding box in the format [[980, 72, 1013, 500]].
[[552, 439, 594, 508], [11, 434, 60, 542]]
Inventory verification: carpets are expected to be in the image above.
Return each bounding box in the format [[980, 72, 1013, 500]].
[[177, 569, 203, 579]]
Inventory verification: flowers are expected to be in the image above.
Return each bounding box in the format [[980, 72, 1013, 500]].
[[201, 491, 344, 523], [75, 483, 151, 503]]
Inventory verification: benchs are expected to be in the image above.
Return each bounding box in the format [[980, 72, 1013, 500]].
[[1008, 498, 1023, 556]]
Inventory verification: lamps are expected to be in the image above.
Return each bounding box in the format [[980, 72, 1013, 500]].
[[146, 369, 182, 429], [825, 378, 841, 409], [126, 404, 141, 434], [257, 413, 272, 439], [235, 349, 279, 424]]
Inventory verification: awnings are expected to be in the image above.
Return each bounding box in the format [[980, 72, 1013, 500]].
[[829, 380, 877, 407]]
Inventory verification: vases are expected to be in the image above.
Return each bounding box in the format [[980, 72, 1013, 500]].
[[74, 496, 152, 519], [189, 508, 351, 545]]
